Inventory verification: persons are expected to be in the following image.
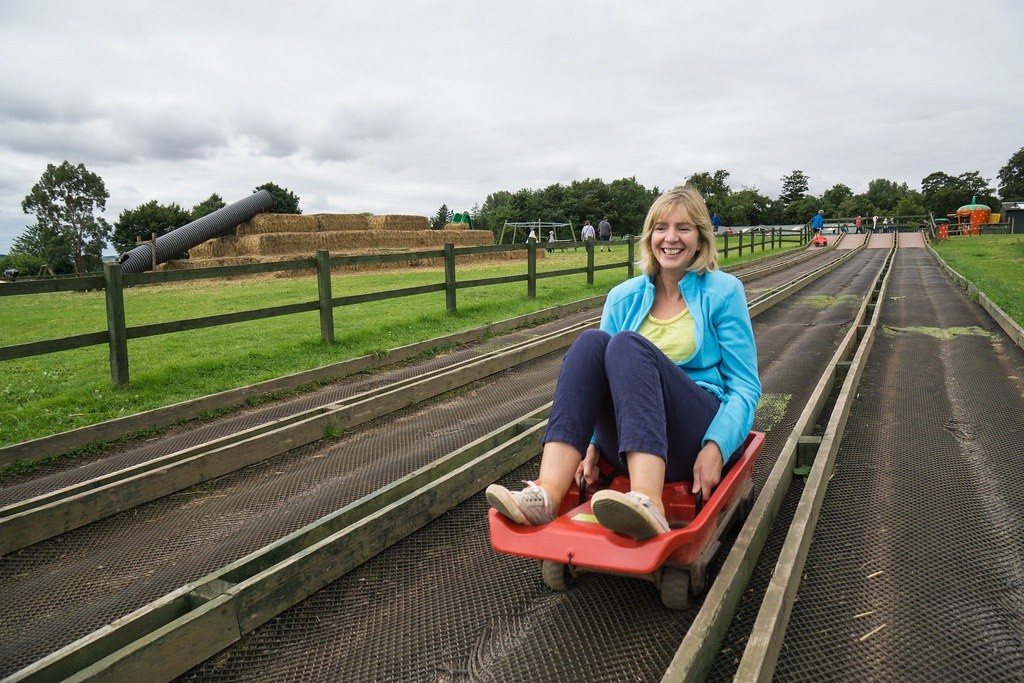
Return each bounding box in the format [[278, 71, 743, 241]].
[[855, 215, 863, 234], [883, 218, 888, 233], [841, 224, 848, 234], [547, 231, 554, 253], [485, 188, 763, 541], [918, 219, 928, 230], [581, 221, 595, 252], [811, 210, 824, 243], [888, 218, 894, 233], [598, 217, 612, 252]]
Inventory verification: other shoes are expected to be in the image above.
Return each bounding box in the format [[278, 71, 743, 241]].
[[601, 249, 603, 252], [608, 249, 611, 252]]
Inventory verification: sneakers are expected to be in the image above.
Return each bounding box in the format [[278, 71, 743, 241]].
[[485, 479, 557, 526], [591, 488, 671, 541]]
